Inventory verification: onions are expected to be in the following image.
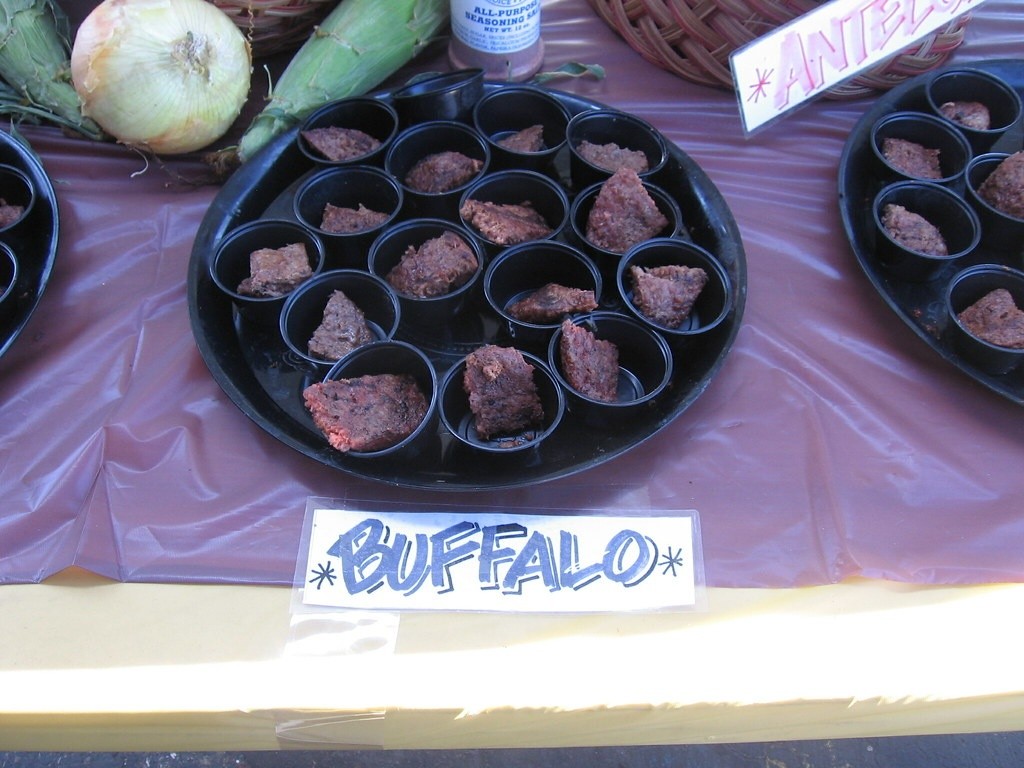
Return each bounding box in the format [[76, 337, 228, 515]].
[[71, 0, 252, 155]]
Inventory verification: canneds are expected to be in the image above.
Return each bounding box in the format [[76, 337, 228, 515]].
[[446, 0, 546, 85]]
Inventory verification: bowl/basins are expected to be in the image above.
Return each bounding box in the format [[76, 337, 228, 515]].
[[945, 263, 1024, 376], [280, 268, 401, 373], [385, 119, 496, 200], [296, 96, 399, 173], [483, 240, 602, 345], [570, 182, 683, 256], [391, 68, 485, 124], [0, 164, 36, 233], [472, 86, 572, 169], [0, 241, 19, 303], [548, 311, 673, 431], [616, 238, 734, 341], [209, 217, 326, 325], [292, 165, 405, 241], [869, 111, 973, 189], [964, 153, 1024, 252], [459, 168, 570, 262], [872, 180, 983, 282], [926, 67, 1023, 154], [440, 349, 565, 452], [367, 219, 485, 327], [566, 109, 669, 187], [324, 341, 439, 462]]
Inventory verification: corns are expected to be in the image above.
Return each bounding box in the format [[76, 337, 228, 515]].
[[205, 0, 449, 175], [0, 0, 108, 142]]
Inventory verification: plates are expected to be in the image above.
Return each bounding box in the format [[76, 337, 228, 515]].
[[0, 130, 60, 357], [838, 59, 1024, 408], [186, 80, 748, 493]]
